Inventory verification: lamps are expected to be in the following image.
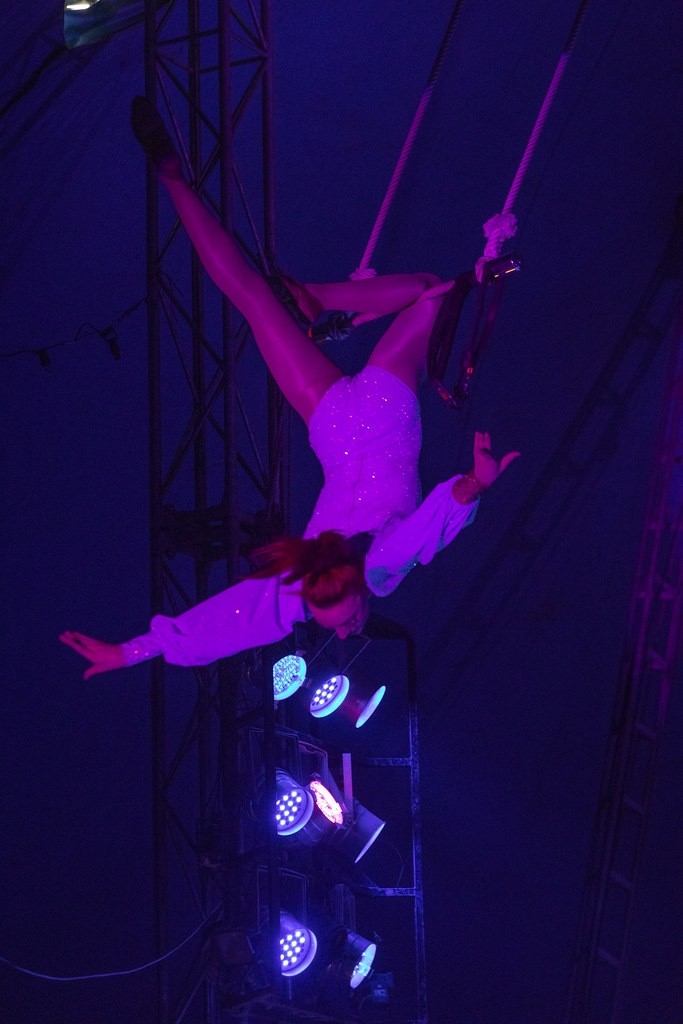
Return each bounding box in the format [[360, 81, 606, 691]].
[[247, 646, 389, 989]]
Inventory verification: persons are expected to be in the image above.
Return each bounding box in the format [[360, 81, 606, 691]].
[[60, 94, 521, 679]]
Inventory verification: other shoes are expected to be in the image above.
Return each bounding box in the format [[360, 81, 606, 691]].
[[270, 254, 322, 331], [132, 95, 183, 179]]
